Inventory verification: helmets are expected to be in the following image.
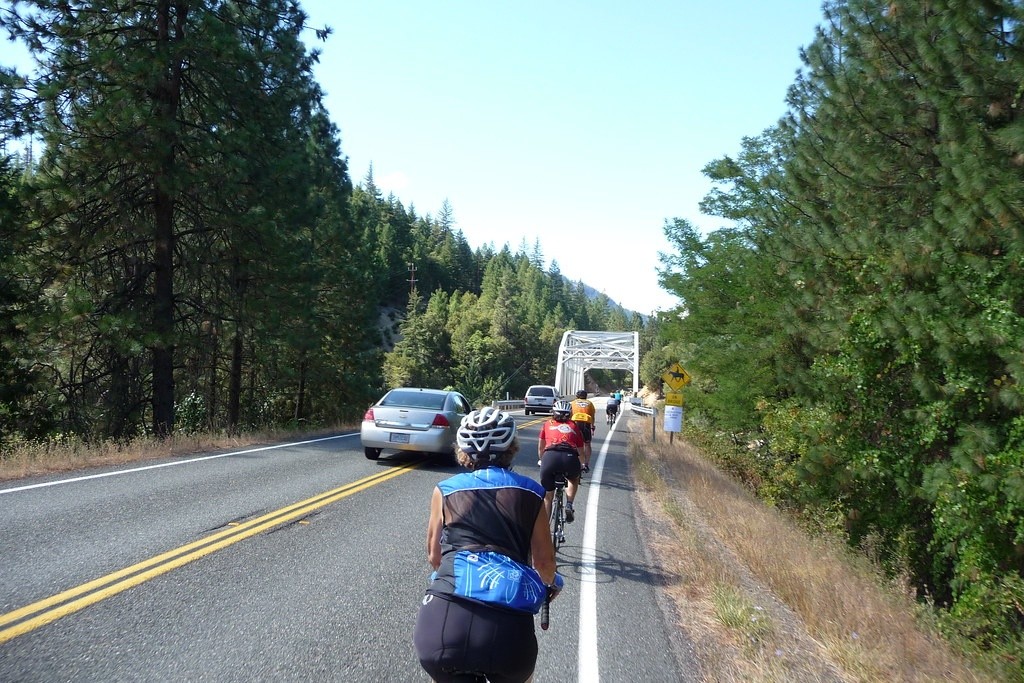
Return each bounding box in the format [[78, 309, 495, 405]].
[[456, 407, 515, 462], [576, 390, 587, 399], [610, 392, 615, 398], [553, 400, 572, 420]]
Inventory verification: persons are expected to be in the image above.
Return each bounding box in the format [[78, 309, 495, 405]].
[[414, 407, 564, 683], [539, 400, 586, 524], [569, 390, 595, 473], [606, 389, 623, 424]]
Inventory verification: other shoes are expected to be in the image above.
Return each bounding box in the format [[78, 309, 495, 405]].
[[584, 466, 589, 473], [566, 505, 574, 524]]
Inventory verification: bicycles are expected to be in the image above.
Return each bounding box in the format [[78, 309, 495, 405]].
[[538, 464, 588, 551], [607, 409, 616, 427], [443, 586, 549, 683]]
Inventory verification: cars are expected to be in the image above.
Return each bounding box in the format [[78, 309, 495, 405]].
[[360, 387, 478, 459], [524, 385, 565, 415]]
